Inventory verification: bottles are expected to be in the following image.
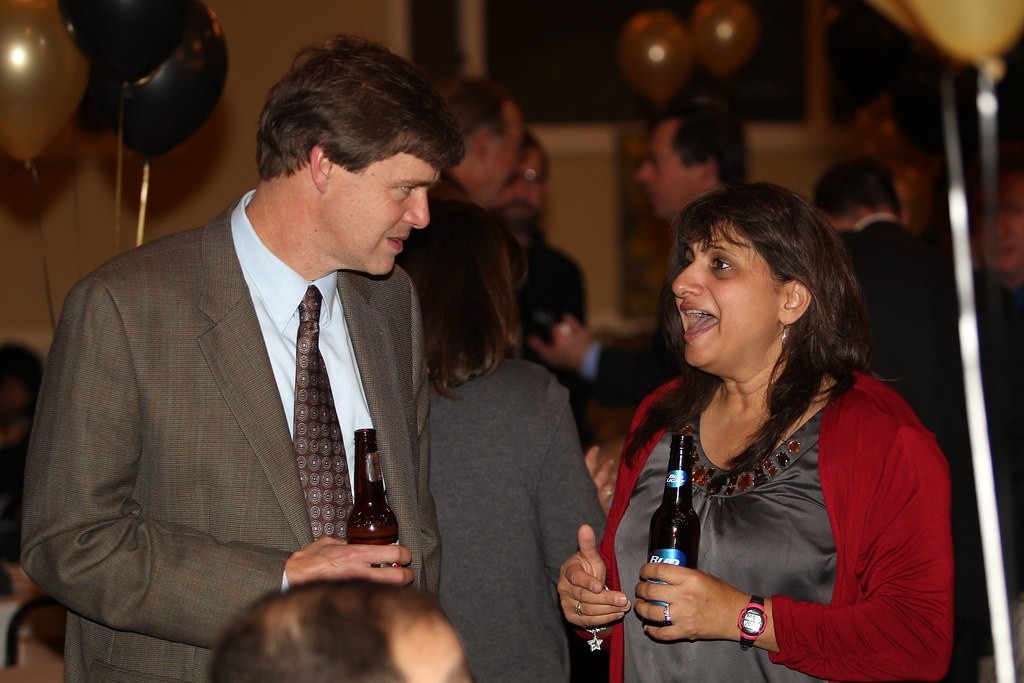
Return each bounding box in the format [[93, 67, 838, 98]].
[[642, 435, 700, 627], [346, 429, 402, 569]]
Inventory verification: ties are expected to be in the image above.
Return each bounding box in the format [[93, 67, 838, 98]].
[[293, 285, 355, 546]]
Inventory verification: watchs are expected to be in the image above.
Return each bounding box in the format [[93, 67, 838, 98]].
[[738, 595, 767, 651]]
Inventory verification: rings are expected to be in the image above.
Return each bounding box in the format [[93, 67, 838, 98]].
[[576, 602, 583, 615], [605, 489, 614, 497], [663, 605, 671, 621], [578, 588, 587, 602]]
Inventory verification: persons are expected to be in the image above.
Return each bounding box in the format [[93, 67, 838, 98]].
[[556, 183, 955, 683], [491, 128, 598, 458], [395, 200, 610, 683], [208, 579, 476, 683], [973, 150, 1024, 683], [0, 339, 56, 593], [420, 72, 523, 210], [814, 153, 991, 683], [531, 101, 750, 411], [20, 33, 463, 683]]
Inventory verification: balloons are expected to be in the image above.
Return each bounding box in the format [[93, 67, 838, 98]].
[[866, 0, 1023, 87], [691, 0, 759, 84], [0, 0, 229, 161], [619, 8, 691, 110]]
